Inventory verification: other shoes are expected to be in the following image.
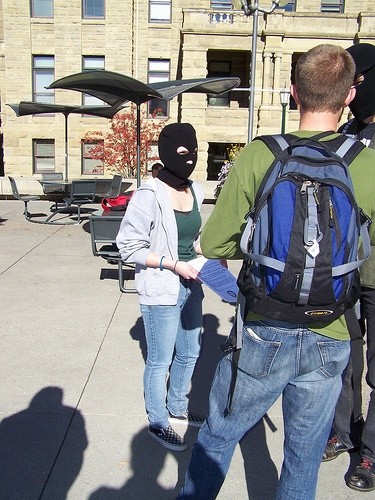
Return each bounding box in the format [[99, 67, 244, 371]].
[[148, 425, 187, 452], [170, 415, 207, 427]]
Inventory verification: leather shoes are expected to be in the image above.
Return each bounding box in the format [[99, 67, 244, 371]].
[[347, 457, 375, 492], [322, 435, 362, 462]]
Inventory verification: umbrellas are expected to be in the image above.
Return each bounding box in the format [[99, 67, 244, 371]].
[[44, 70, 241, 188], [6, 100, 129, 182]]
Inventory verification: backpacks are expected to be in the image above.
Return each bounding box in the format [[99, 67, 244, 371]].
[[236, 134, 373, 327]]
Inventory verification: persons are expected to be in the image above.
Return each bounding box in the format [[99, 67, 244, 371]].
[[321, 44, 375, 491], [177, 44, 375, 500], [116, 120, 228, 451]]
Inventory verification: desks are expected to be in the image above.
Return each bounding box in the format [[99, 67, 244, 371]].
[[39, 179, 112, 230]]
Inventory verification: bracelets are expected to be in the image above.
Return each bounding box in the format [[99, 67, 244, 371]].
[[174, 260, 178, 271], [160, 256, 165, 271]]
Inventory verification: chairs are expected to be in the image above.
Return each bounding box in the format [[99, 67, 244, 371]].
[[8, 173, 140, 293]]
